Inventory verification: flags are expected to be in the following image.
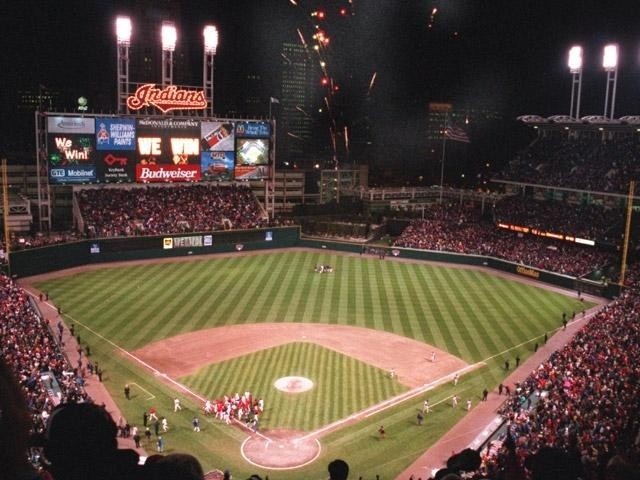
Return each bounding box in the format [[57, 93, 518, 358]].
[[444, 114, 472, 143]]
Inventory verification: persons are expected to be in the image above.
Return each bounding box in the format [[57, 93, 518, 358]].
[[378, 425, 385, 439], [434, 292, 639, 480], [417, 412, 423, 425], [1, 131, 639, 291], [328, 458, 350, 480], [429, 408, 433, 417], [1, 291, 266, 478], [430, 352, 436, 363], [389, 367, 395, 380], [423, 399, 430, 414]]
